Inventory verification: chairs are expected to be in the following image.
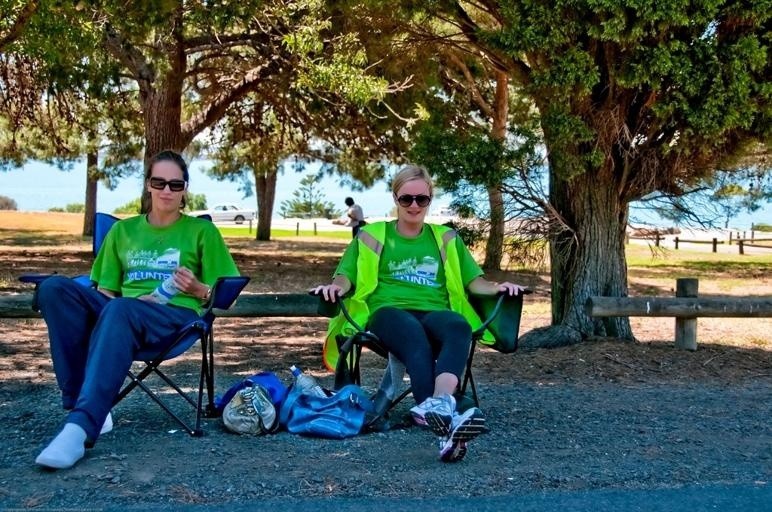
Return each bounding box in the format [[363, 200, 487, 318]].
[[17, 212, 248, 443], [307, 289, 534, 433]]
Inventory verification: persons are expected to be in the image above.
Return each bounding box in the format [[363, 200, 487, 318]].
[[35, 150, 241, 469], [344, 197, 363, 238], [310, 164, 529, 462]]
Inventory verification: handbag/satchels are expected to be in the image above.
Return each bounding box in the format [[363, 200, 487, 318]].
[[223, 384, 280, 438], [281, 380, 375, 439]]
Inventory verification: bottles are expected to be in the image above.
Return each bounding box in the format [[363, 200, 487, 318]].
[[151, 268, 188, 305], [288, 365, 327, 401]]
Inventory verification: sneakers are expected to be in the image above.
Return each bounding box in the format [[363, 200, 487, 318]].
[[35, 423, 87, 469], [99, 413, 113, 435], [409, 393, 457, 437], [437, 407, 484, 463]]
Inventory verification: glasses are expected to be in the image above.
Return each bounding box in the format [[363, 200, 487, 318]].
[[394, 192, 432, 208], [149, 176, 188, 192]]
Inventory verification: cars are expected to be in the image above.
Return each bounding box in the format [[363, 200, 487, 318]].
[[189, 204, 254, 224]]
